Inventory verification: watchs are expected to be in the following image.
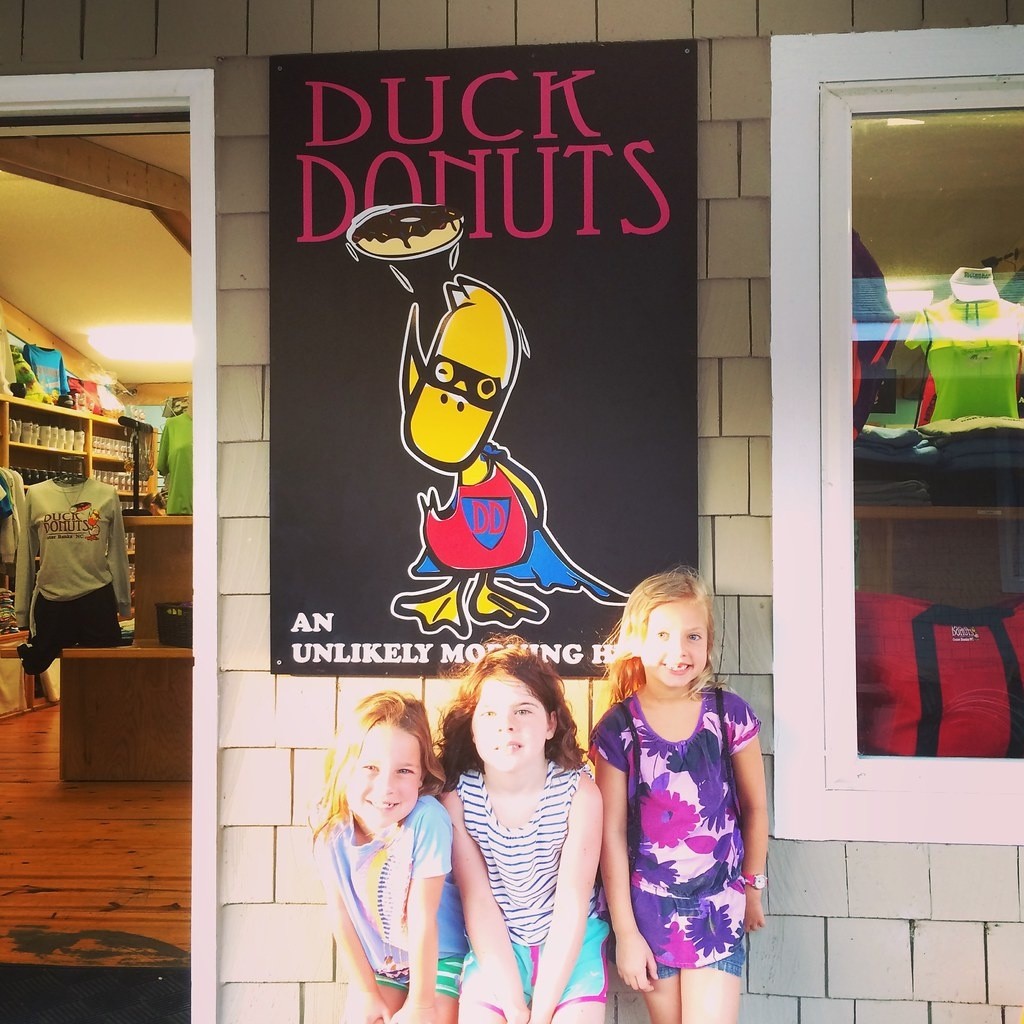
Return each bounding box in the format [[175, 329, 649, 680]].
[[743, 873, 767, 889]]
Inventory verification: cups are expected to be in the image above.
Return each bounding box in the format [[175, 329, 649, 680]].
[[75, 393, 95, 414], [9, 418, 85, 452], [11, 466, 82, 486]]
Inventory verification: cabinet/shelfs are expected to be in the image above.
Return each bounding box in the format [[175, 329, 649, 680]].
[[0, 515, 195, 784], [0, 394, 160, 582]]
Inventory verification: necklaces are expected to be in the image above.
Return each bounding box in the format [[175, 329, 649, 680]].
[[58, 479, 85, 513]]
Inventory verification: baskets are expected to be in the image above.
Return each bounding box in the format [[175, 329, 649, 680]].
[[155, 602, 192, 648]]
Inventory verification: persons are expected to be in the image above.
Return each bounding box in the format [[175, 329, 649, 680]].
[[156, 397, 193, 516], [436, 650, 613, 1024], [312, 690, 469, 1024], [16, 455, 133, 674], [906, 266, 1024, 422], [587, 574, 769, 1024]]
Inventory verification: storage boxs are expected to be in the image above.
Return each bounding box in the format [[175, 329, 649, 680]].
[[86, 396, 96, 413], [71, 393, 86, 412]]
